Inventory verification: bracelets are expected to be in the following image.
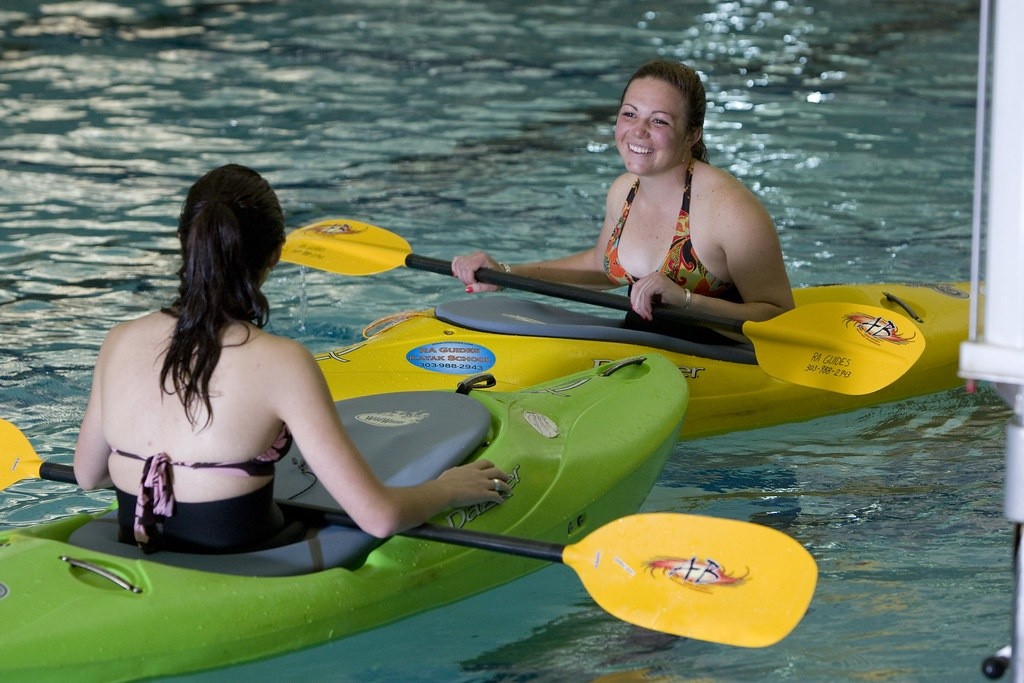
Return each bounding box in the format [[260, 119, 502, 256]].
[[499, 262, 511, 290], [684, 288, 691, 311]]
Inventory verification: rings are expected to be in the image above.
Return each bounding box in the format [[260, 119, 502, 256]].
[[493, 478, 499, 490]]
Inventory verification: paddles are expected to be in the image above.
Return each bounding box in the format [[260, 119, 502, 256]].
[[0, 418, 820, 649], [278, 219, 926, 396]]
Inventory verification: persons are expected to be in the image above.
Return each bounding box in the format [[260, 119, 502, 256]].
[[73, 163, 512, 554], [451, 60, 795, 346]]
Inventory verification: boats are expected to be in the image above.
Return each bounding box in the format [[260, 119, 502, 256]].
[[310, 279, 985, 442], [1, 351, 690, 682]]
